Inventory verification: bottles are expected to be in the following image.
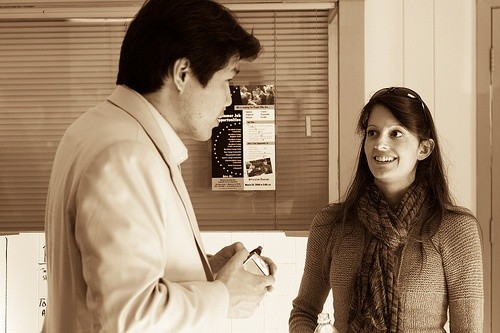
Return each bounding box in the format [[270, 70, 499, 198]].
[[313, 313, 339, 333]]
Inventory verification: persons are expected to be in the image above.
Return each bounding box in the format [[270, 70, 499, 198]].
[[289, 86, 485, 332], [37, 0, 277, 332]]
